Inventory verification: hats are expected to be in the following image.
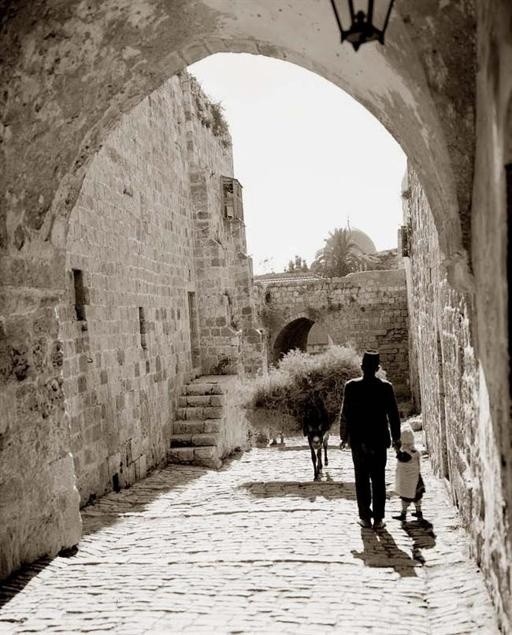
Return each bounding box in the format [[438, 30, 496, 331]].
[[362, 349, 380, 368]]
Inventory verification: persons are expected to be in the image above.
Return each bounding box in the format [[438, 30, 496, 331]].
[[391, 422, 427, 523], [337, 348, 404, 531]]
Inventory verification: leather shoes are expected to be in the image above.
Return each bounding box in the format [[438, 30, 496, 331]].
[[411, 511, 423, 517], [393, 513, 406, 519], [372, 519, 386, 529], [357, 515, 371, 528]]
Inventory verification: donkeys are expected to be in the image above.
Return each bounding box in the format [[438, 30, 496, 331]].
[[299, 397, 333, 479]]
